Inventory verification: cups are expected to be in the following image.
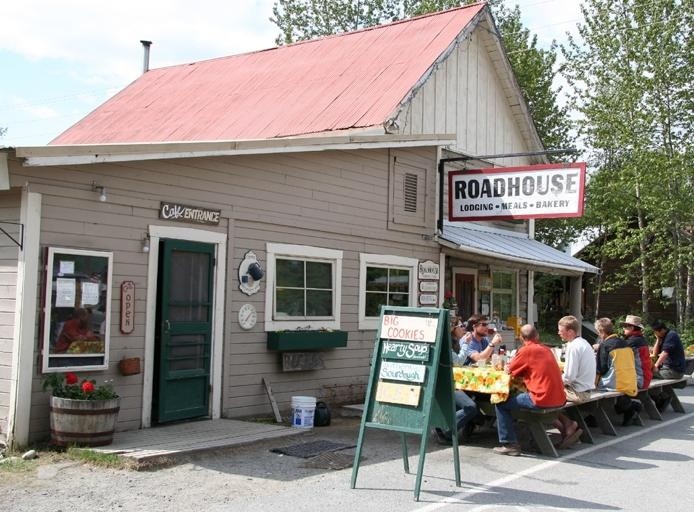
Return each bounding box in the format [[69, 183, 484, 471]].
[[478, 360, 486, 366]]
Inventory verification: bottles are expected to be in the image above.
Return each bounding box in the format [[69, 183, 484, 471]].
[[551, 343, 566, 364], [491, 347, 510, 371]]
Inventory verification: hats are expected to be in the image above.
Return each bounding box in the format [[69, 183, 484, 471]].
[[620, 315, 644, 330]]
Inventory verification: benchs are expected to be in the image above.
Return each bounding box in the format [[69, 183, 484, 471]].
[[511, 376, 690, 459]]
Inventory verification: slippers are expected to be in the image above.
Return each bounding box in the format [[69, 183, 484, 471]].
[[556, 428, 583, 449]]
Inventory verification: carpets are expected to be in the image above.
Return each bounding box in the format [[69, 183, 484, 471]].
[[268, 436, 358, 458], [300, 447, 366, 471]]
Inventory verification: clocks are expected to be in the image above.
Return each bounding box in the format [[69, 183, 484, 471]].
[[236, 301, 259, 330]]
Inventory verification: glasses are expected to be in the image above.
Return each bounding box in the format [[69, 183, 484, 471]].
[[477, 323, 488, 326], [455, 324, 465, 328]]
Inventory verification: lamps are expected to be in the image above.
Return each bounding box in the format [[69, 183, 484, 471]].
[[90, 179, 107, 203], [139, 231, 150, 254]]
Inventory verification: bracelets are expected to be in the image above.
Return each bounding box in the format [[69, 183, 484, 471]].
[[488, 342, 495, 349]]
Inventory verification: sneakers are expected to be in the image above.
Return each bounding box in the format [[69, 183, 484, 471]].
[[493, 446, 519, 456], [655, 391, 673, 414], [622, 401, 643, 427]]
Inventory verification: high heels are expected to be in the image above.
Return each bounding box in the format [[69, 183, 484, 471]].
[[431, 427, 449, 445]]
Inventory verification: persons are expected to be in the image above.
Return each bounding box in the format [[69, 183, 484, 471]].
[[547, 315, 597, 449], [459, 313, 503, 367], [618, 314, 653, 426], [492, 324, 567, 456], [431, 316, 481, 443], [649, 322, 686, 413], [584, 317, 639, 426], [54, 272, 105, 351]]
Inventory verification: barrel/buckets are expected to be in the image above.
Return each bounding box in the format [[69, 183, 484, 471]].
[[119, 349, 141, 376], [49, 396, 121, 447], [291, 395, 317, 427]]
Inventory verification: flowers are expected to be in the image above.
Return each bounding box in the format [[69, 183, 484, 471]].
[[42, 370, 123, 403], [442, 289, 456, 309], [454, 370, 503, 393]]
